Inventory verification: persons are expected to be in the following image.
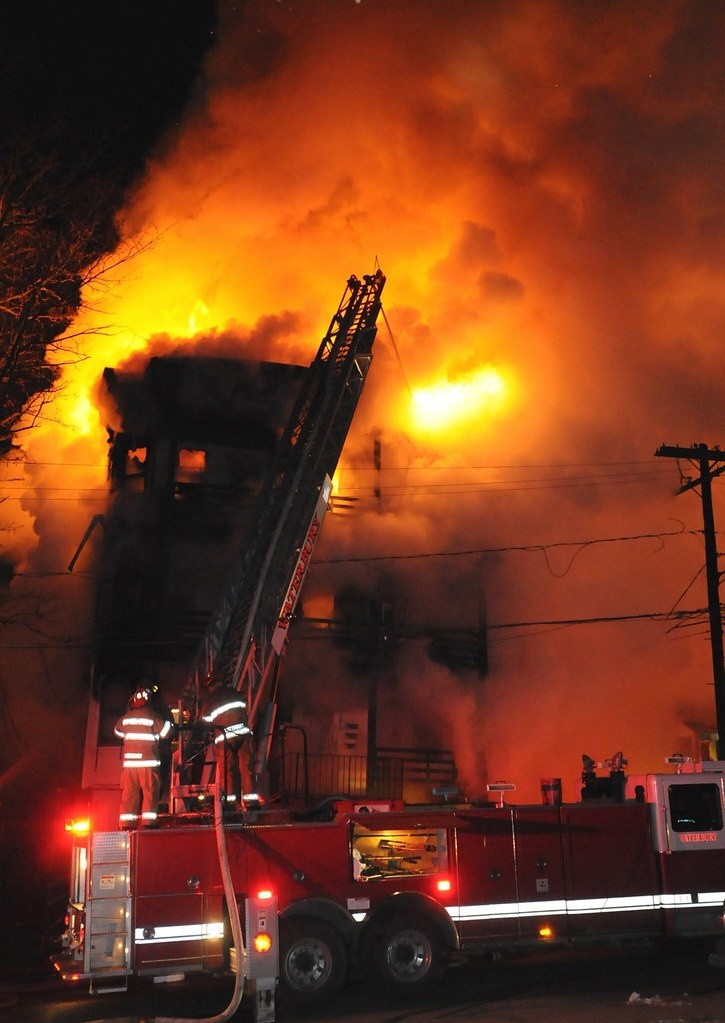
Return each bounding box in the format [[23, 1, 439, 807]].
[[198, 669, 256, 826], [112, 685, 179, 830]]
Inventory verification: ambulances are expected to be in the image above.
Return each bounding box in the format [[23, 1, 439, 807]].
[[50, 269, 724, 1023]]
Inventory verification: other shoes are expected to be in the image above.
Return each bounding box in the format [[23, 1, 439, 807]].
[[221, 804, 236, 812], [143, 823, 161, 830], [121, 825, 137, 831], [247, 804, 261, 811]]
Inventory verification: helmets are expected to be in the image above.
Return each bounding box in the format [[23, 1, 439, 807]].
[[129, 687, 154, 709], [203, 674, 221, 688]]
